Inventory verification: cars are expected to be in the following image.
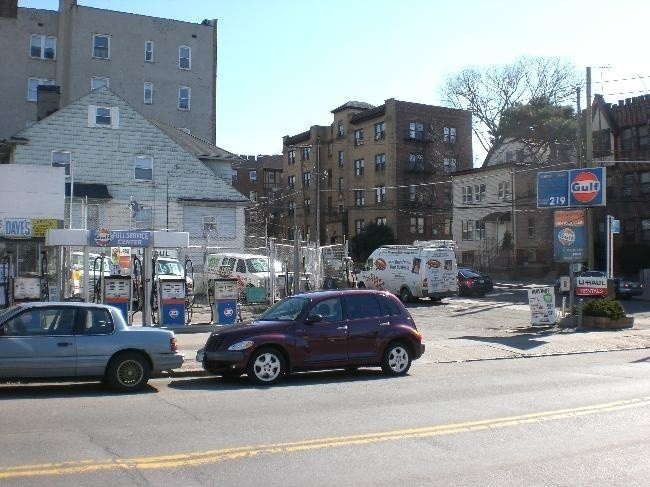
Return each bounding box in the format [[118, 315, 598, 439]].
[[551, 269, 643, 299], [196, 289, 427, 384], [322, 238, 495, 304], [0, 301, 186, 392]]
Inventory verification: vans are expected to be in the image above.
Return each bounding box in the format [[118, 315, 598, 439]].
[[71, 249, 296, 304]]
